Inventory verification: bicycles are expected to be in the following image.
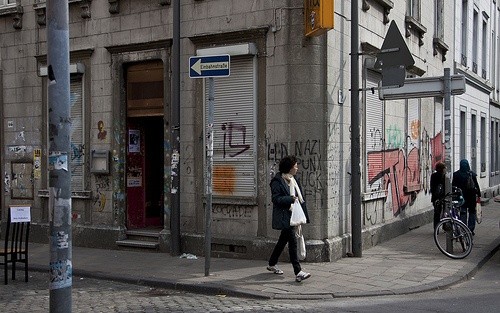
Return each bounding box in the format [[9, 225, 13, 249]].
[[433, 187, 474, 259]]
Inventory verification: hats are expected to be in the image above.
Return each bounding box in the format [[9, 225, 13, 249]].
[[434, 161, 448, 170]]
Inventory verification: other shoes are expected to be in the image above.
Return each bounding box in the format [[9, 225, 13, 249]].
[[434, 231, 439, 235]]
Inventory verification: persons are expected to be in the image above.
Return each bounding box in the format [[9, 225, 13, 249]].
[[266, 155, 311, 282], [452, 159, 482, 235], [430, 163, 451, 235], [98, 120, 107, 140]]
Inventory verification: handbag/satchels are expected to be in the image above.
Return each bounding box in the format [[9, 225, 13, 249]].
[[467, 170, 478, 195], [475, 197, 483, 224], [287, 225, 306, 261], [289, 198, 307, 226]]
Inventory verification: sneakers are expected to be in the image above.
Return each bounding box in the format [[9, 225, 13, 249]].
[[472, 232, 474, 235], [296, 271, 311, 282], [267, 265, 283, 274]]
